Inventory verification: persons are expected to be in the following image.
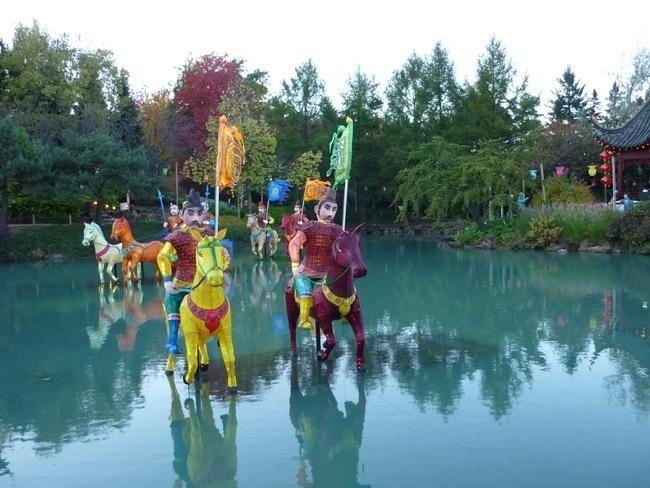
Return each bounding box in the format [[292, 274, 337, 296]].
[[292, 201, 309, 228], [615, 190, 634, 214], [163, 201, 186, 232], [517, 192, 529, 209], [605, 180, 650, 205], [156, 188, 220, 354], [201, 202, 214, 227], [257, 203, 274, 238], [287, 188, 341, 329]]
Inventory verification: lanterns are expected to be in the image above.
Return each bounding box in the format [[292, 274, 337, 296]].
[[597, 149, 614, 189], [528, 169, 539, 181], [555, 166, 564, 176], [586, 165, 597, 176]]
